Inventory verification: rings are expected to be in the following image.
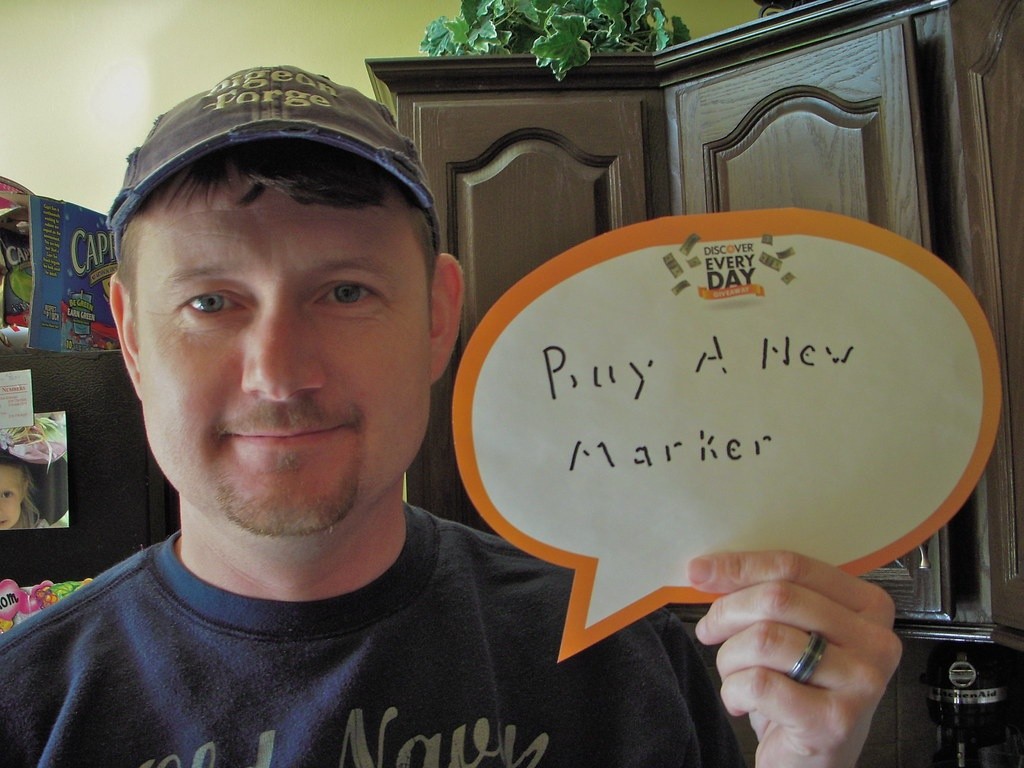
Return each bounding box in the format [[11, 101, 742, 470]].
[[786, 629, 828, 682]]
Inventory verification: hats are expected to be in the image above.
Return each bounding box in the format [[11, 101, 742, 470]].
[[106, 65, 442, 263]]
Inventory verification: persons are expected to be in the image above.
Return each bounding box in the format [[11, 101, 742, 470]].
[[0, 63, 901, 768], [0, 418, 69, 527], [0, 453, 50, 530]]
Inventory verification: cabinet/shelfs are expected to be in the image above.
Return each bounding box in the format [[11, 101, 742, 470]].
[[360, 0, 1024, 768]]
